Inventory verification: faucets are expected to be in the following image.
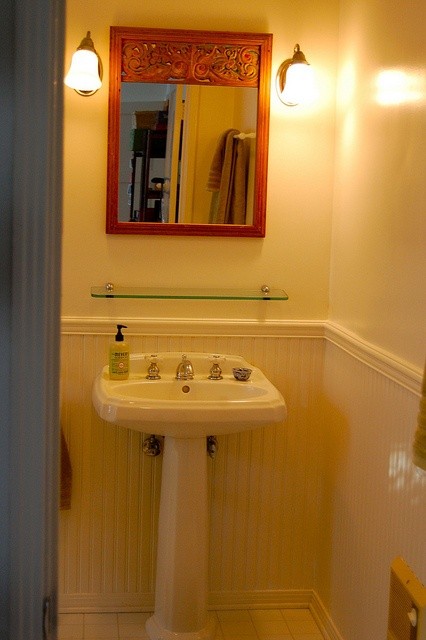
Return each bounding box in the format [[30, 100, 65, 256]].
[[174, 360, 194, 382]]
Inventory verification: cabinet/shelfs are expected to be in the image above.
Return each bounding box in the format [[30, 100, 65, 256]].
[[129, 128, 166, 222]]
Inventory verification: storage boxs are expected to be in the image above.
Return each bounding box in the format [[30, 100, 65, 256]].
[[134, 109, 167, 131]]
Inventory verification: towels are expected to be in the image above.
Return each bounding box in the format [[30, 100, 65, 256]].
[[205, 127, 246, 225]]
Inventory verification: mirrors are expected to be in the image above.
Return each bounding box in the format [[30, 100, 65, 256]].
[[102, 25, 272, 238]]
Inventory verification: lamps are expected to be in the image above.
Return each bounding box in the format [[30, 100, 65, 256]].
[[62, 30, 103, 97], [275, 44, 319, 107]]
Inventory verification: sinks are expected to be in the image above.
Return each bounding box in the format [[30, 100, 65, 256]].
[[91, 353, 286, 437]]
